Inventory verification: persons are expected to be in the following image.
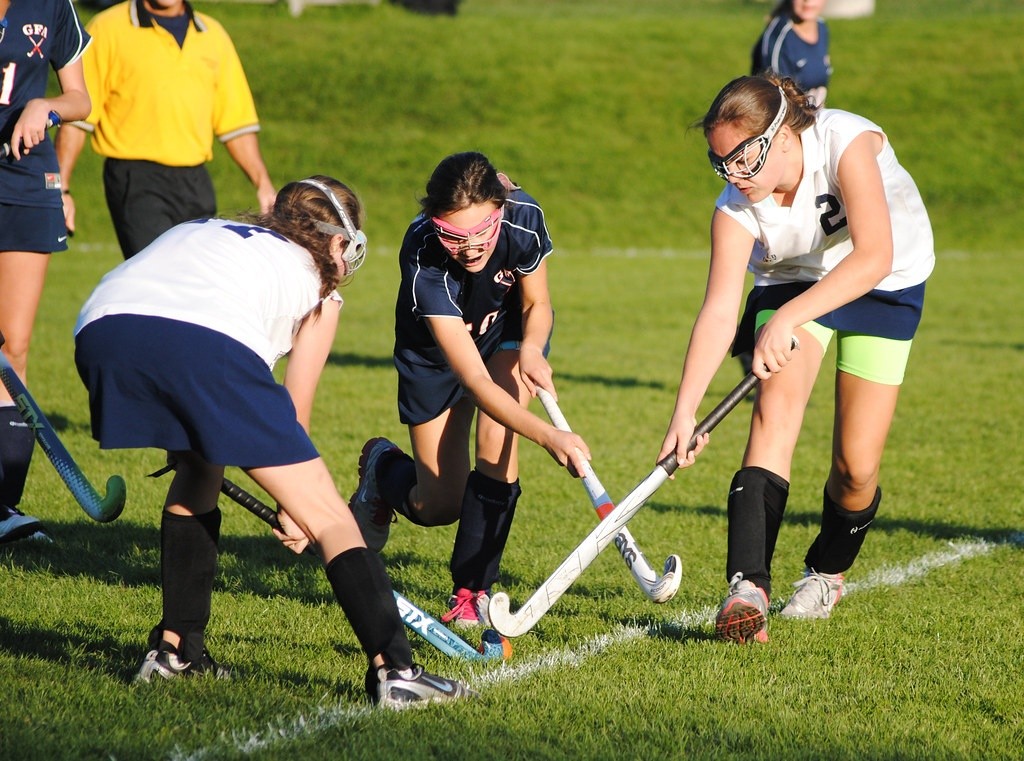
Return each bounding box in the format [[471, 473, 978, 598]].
[[749, 0, 831, 400], [348, 152, 591, 629], [656, 76, 936, 642], [0, 0, 279, 542], [74, 175, 482, 710]]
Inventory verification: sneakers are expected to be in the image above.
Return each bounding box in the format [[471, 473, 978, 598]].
[[348, 437, 403, 551], [715, 571, 768, 643], [0, 504, 52, 542], [366, 663, 480, 711], [133, 650, 229, 688], [442, 587, 491, 630], [781, 567, 843, 618]]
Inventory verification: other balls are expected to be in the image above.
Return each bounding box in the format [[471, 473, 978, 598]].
[[477, 635, 513, 662]]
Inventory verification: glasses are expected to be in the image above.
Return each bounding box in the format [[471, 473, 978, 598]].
[[708, 135, 771, 181], [431, 209, 501, 254], [342, 230, 368, 276]]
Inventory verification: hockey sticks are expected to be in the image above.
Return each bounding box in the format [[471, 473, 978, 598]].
[[488, 333, 800, 638], [529, 386, 682, 605], [0, 109, 63, 159], [0, 351, 128, 523], [167, 456, 504, 669]]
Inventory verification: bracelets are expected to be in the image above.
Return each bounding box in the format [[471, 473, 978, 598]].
[[26, 98, 57, 117]]
